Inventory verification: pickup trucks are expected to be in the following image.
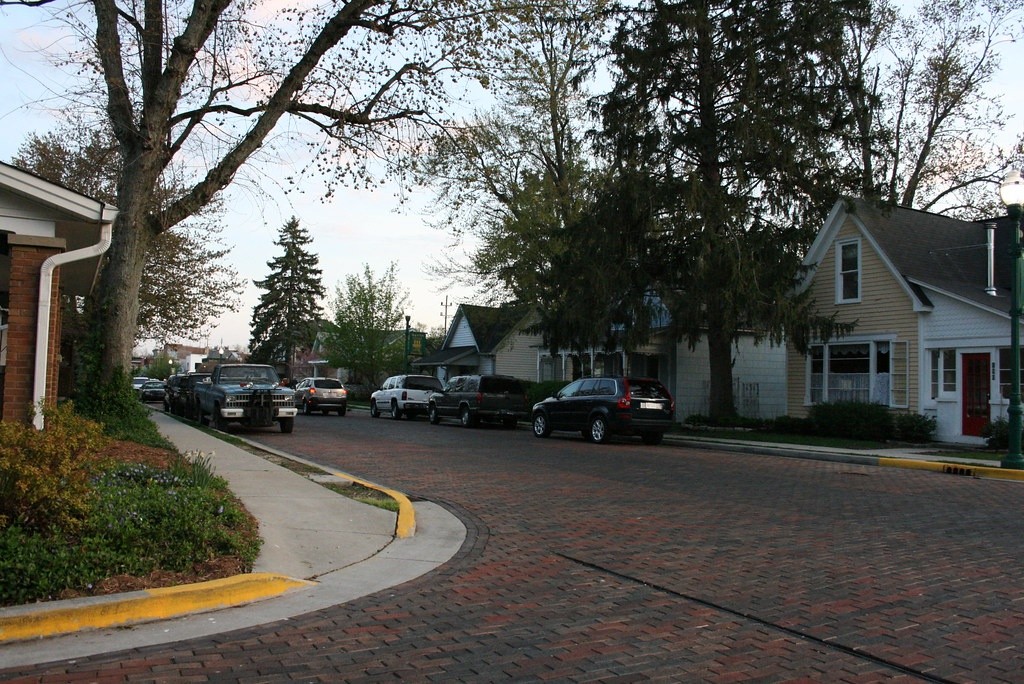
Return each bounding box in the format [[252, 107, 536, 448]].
[[195, 363, 298, 433]]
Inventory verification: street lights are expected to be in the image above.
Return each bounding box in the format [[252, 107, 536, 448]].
[[1001, 169, 1024, 470], [404, 316, 411, 373], [219, 348, 225, 364]]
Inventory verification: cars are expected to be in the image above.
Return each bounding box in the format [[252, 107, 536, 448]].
[[164, 373, 213, 419], [131, 377, 149, 394], [139, 381, 165, 403]]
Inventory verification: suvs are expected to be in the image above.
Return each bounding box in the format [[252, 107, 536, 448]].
[[428, 374, 530, 429], [371, 374, 443, 419], [531, 373, 677, 447], [295, 377, 348, 416]]
